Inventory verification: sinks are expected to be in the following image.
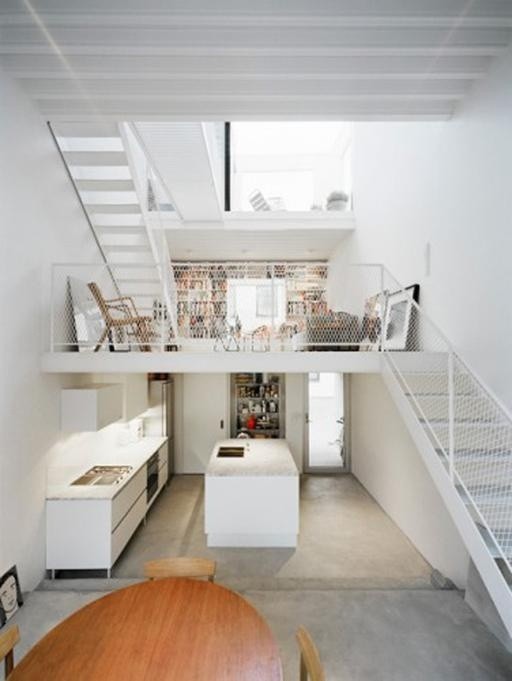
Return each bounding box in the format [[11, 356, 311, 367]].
[[216, 447, 244, 457]]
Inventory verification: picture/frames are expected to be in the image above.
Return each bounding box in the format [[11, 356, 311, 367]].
[[380, 283, 420, 351], [106, 306, 132, 352]]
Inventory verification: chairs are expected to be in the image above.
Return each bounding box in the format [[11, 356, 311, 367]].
[[0, 624, 21, 681], [209, 314, 240, 352], [87, 281, 153, 353], [294, 624, 327, 681], [142, 556, 217, 583]]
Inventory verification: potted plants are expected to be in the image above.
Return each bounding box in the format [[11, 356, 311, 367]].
[[325, 190, 349, 211]]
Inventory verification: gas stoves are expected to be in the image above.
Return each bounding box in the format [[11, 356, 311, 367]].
[[70, 465, 133, 487]]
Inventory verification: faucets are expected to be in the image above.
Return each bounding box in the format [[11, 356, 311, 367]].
[[237, 433, 249, 446]]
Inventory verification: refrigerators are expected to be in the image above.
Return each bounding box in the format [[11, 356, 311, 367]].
[[150, 380, 176, 480]]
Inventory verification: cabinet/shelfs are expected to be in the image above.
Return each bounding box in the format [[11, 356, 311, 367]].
[[45, 464, 147, 580], [158, 440, 168, 494], [60, 383, 121, 433], [170, 260, 328, 340], [230, 373, 286, 440]]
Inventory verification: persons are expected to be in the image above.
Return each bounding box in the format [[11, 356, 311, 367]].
[[1, 575, 22, 624]]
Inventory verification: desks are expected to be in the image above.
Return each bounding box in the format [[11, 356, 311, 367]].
[[4, 575, 285, 680]]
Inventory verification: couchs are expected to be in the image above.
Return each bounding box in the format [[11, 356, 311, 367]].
[[291, 312, 359, 351]]
